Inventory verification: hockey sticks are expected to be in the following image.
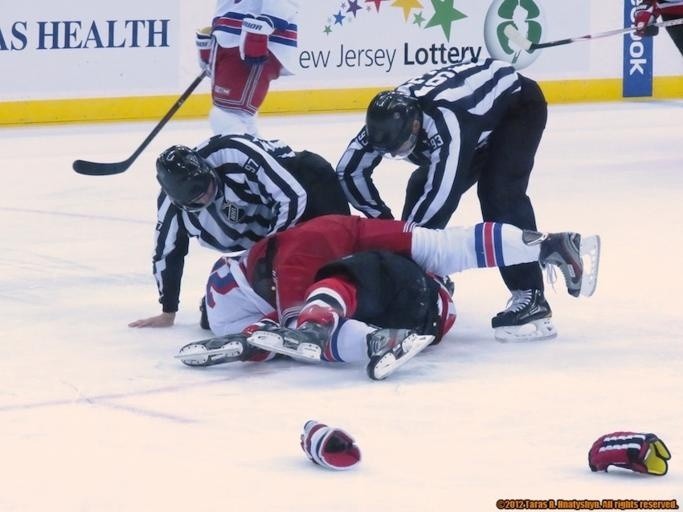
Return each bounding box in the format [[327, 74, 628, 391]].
[[504, 19, 681, 51]]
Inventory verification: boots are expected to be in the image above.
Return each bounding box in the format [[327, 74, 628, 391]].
[[491, 288, 551, 328], [260, 320, 334, 362], [178, 332, 259, 367], [521, 228, 582, 298], [365, 322, 416, 380]]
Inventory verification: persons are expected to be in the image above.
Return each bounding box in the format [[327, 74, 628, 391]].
[[336, 56, 554, 326], [194, 0, 301, 134], [174, 251, 458, 368], [634, 0, 682, 55], [201, 213, 581, 330], [128, 132, 351, 329]]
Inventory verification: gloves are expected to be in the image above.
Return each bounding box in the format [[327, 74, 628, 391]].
[[195, 30, 212, 69], [299, 420, 361, 472], [635, 4, 657, 37], [238, 12, 275, 64], [587, 432, 671, 476]]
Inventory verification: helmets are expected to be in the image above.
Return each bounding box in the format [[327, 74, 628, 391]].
[[364, 90, 423, 161], [155, 145, 221, 213]]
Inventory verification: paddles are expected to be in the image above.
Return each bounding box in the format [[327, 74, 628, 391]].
[[73, 69, 207, 176]]
